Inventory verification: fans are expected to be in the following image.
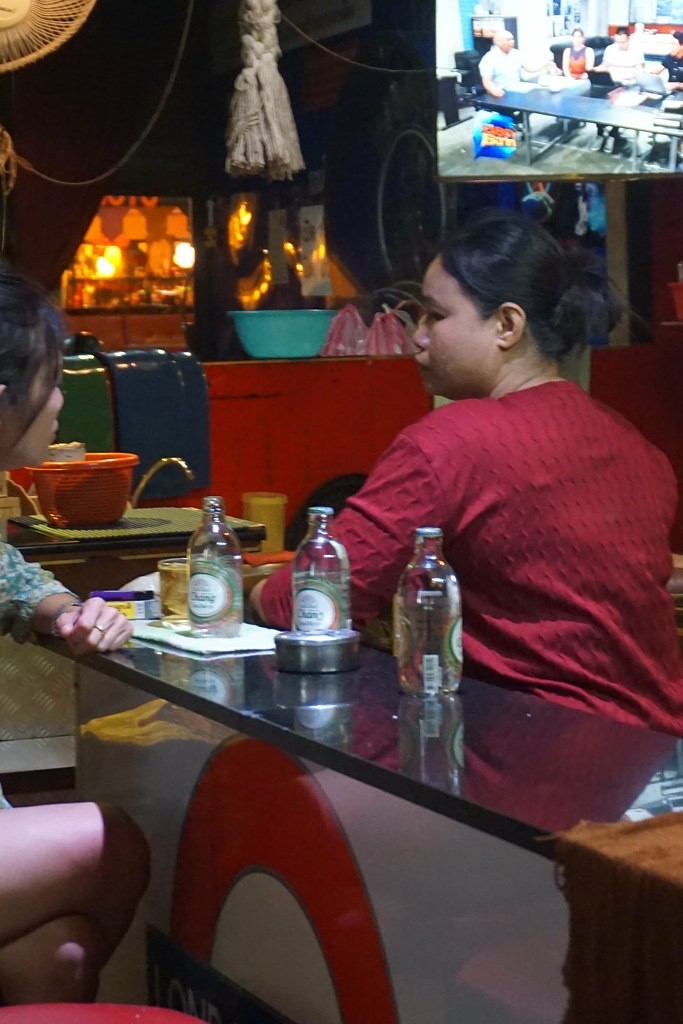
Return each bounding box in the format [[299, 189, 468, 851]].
[[0, 0, 97, 74]]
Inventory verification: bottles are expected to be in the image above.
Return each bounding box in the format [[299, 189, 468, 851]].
[[187, 493, 244, 640], [291, 505, 352, 638], [392, 528, 464, 696]]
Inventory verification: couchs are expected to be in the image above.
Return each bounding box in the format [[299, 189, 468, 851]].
[[552, 36, 614, 72]]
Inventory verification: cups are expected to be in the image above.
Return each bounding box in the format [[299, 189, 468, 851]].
[[158, 556, 191, 627]]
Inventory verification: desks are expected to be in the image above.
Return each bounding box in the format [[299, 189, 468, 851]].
[[475, 84, 683, 173]]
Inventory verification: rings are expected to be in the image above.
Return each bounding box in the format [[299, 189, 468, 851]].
[[93, 625, 106, 637]]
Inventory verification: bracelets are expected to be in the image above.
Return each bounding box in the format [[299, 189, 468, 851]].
[[49, 600, 82, 637]]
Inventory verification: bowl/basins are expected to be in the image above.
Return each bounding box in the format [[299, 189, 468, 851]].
[[23, 451, 141, 530], [226, 310, 339, 359]]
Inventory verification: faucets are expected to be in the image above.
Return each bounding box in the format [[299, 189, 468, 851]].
[[130, 457, 194, 508]]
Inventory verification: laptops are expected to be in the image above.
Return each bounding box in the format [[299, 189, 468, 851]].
[[585, 69, 620, 88], [637, 73, 676, 96]]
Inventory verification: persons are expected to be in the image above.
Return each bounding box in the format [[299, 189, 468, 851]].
[[246, 213, 683, 738], [0, 270, 157, 1010], [477, 27, 683, 142]]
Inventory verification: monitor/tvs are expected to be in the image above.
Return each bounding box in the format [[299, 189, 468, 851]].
[[435, 0, 683, 183]]
